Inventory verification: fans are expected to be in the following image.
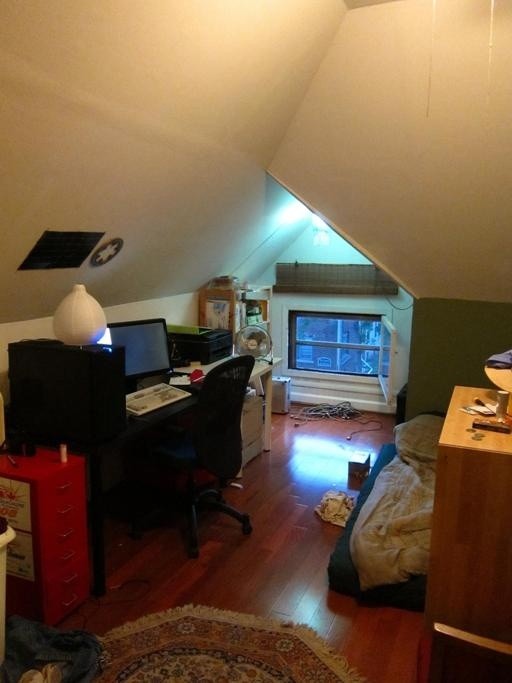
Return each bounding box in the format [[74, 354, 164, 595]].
[[235, 324, 272, 360]]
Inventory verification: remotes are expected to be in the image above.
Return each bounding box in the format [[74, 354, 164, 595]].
[[472, 418, 510, 433]]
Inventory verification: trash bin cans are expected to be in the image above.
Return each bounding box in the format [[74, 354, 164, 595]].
[[0, 527, 16, 665]]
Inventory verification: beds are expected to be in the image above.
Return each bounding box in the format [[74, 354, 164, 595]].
[[326, 438, 437, 611]]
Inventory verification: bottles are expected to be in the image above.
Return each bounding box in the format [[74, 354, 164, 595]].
[[495, 391, 510, 419], [60, 444, 67, 463]]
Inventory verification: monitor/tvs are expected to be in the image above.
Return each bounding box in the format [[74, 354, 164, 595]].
[[97, 318, 171, 382]]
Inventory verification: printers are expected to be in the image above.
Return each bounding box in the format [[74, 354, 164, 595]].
[[166, 323, 234, 365]]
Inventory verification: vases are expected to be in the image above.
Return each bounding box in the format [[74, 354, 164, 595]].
[[54, 283, 108, 346]]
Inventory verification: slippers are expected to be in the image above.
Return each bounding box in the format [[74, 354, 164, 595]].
[[18, 663, 63, 683]]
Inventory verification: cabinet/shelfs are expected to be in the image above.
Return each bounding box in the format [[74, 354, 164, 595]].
[[422, 385, 512, 683], [198, 281, 273, 345], [1, 446, 88, 628]]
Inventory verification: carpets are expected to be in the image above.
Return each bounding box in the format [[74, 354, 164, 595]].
[[54, 602, 367, 682]]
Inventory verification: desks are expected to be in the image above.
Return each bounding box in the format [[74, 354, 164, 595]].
[[121, 354, 284, 480]]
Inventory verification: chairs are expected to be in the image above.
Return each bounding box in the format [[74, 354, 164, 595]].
[[128, 354, 253, 559]]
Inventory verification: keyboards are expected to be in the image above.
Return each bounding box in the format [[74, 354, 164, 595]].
[[125, 382, 192, 417]]
